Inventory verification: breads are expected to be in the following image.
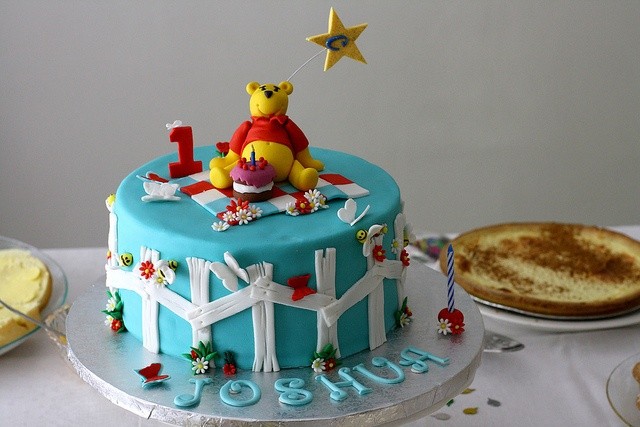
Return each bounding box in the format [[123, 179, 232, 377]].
[[438, 222, 637, 317], [1, 249, 52, 351]]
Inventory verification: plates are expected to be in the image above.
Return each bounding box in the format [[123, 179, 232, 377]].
[[1, 235, 66, 356], [432, 224, 636, 332], [606, 350, 640, 427], [66, 248, 484, 427]]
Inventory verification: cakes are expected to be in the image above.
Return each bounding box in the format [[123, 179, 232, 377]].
[[102, 7, 465, 408]]
